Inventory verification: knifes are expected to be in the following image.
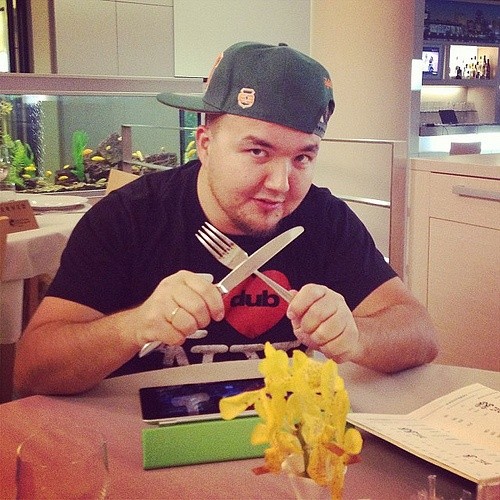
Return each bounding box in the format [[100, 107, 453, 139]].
[[139, 226, 305, 359]]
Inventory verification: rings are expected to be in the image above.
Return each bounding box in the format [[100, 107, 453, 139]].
[[168, 308, 180, 321]]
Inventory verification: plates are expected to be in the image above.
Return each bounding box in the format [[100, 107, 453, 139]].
[[0, 190, 89, 209]]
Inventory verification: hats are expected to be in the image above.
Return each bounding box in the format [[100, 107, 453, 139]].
[[156, 42, 335, 138]]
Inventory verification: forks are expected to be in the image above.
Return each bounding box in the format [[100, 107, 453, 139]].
[[194, 222, 295, 305]]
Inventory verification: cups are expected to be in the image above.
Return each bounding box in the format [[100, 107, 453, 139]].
[[16, 423, 109, 500]]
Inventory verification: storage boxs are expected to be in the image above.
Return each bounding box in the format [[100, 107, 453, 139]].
[[0, 72, 209, 199]]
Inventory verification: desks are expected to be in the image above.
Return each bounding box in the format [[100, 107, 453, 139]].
[[0, 358, 500, 500], [0, 191, 93, 345]]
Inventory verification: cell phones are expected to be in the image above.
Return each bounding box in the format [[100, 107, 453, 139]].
[[139, 377, 265, 424]]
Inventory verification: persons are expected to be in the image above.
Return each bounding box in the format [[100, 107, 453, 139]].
[[11, 40, 439, 398]]
